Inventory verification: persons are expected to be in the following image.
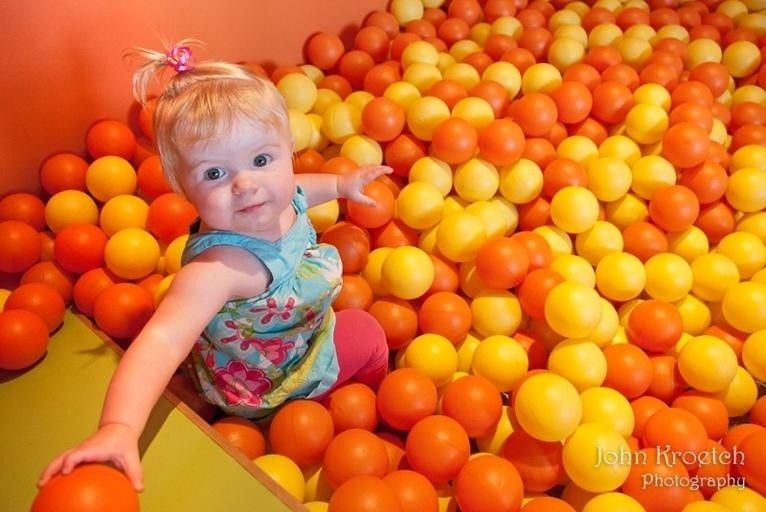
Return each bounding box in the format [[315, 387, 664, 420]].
[[1, 0, 764, 510], [0, 1, 765, 511]]
